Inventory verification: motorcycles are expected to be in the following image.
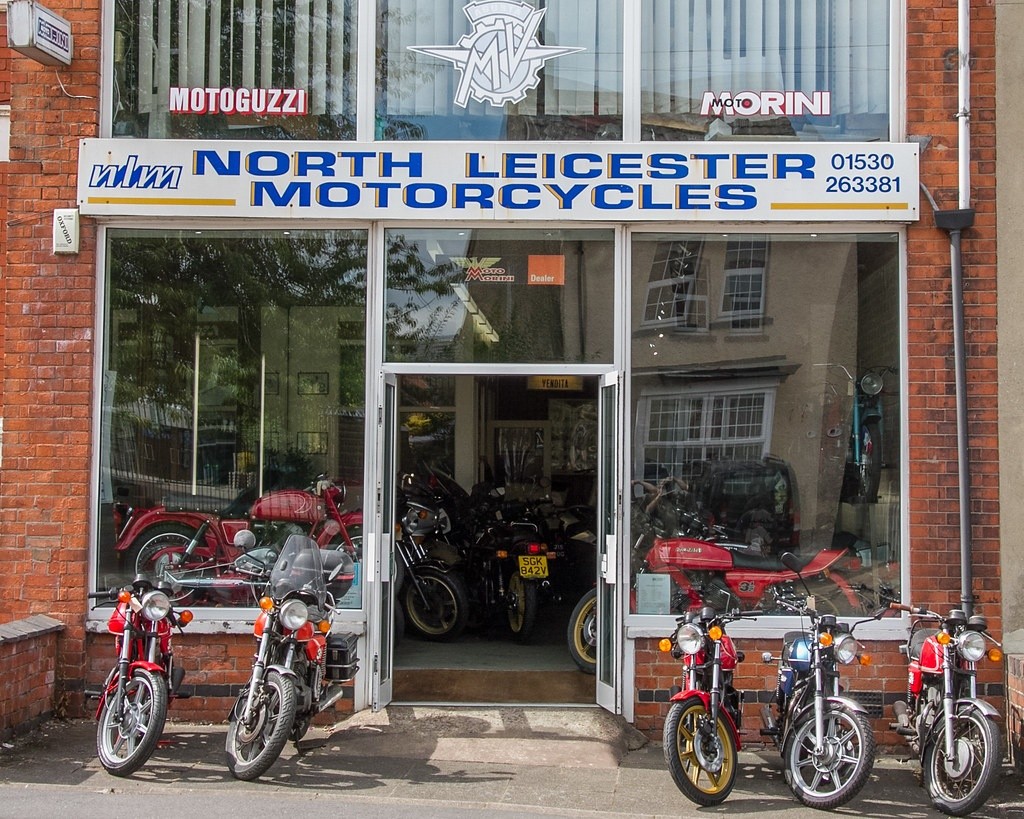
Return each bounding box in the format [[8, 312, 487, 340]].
[[113, 471, 363, 608], [889, 603, 1002, 816], [760, 552, 887, 810], [225, 529, 360, 780], [395, 471, 552, 643], [658, 589, 757, 806], [567, 476, 900, 675], [786, 362, 900, 504], [87, 574, 193, 776]]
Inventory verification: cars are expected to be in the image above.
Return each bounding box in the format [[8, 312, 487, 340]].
[[121, 427, 290, 484], [408, 416, 493, 457], [670, 454, 800, 551]]
